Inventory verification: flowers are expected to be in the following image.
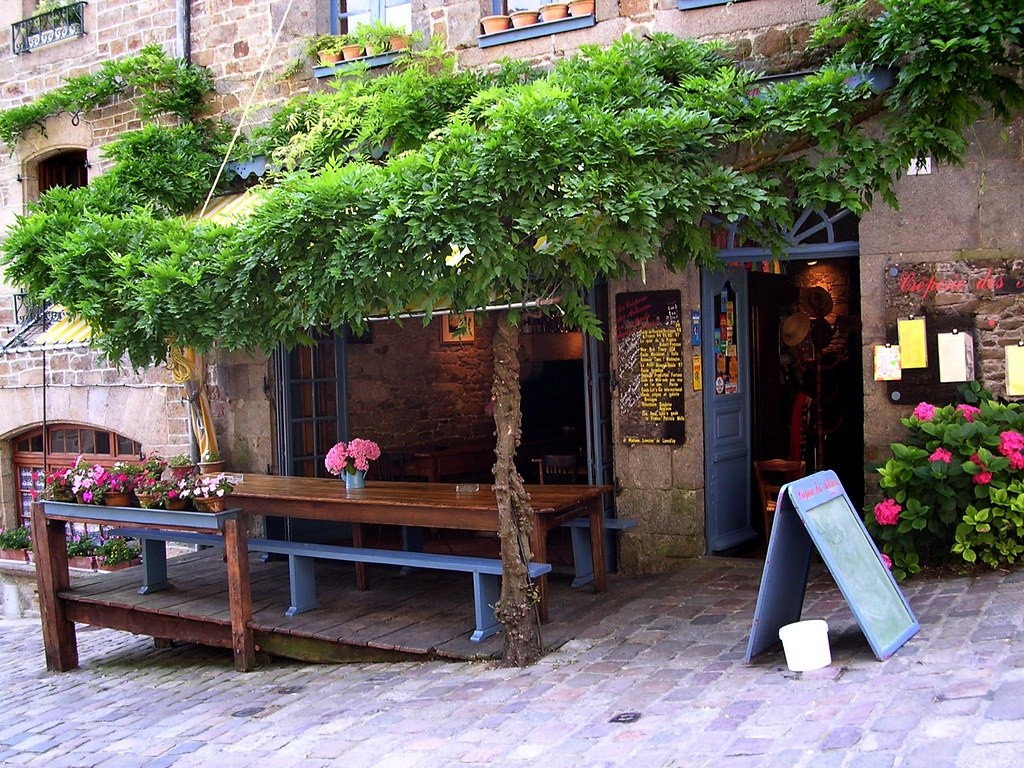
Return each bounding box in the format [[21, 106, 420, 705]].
[[0, 523, 33, 553], [29, 453, 236, 509], [324, 438, 381, 476], [66, 533, 140, 566]]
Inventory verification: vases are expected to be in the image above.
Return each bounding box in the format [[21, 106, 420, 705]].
[[481, 0, 594, 35], [0, 547, 30, 561], [166, 499, 189, 512], [26, 551, 32, 561], [341, 465, 369, 489], [103, 492, 132, 508], [75, 492, 93, 505], [68, 556, 98, 569], [94, 556, 141, 571], [152, 469, 164, 483], [194, 495, 226, 512], [135, 493, 158, 509], [56, 489, 74, 504]]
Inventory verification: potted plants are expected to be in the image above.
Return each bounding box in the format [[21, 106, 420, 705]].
[[198, 453, 226, 475], [16, 0, 82, 53], [260, 21, 412, 85], [168, 453, 198, 480]]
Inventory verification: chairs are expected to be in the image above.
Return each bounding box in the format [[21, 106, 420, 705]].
[[753, 459, 807, 552], [535, 455, 579, 486]]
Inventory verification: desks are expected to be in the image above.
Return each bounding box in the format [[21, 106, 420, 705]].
[[386, 438, 497, 539], [199, 472, 615, 624]]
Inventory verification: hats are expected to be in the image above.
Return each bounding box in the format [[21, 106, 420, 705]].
[[782, 312, 810, 346], [811, 319, 832, 348], [801, 287, 833, 317]]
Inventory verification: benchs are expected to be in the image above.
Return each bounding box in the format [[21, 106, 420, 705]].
[[107, 529, 552, 642], [555, 516, 637, 588]]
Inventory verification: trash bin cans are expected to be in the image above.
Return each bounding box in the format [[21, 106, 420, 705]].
[[2, 581, 22, 620]]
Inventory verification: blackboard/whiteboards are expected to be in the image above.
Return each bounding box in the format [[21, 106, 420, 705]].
[[787, 469, 921, 662]]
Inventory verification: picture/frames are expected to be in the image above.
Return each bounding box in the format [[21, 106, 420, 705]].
[[440, 311, 476, 345]]
